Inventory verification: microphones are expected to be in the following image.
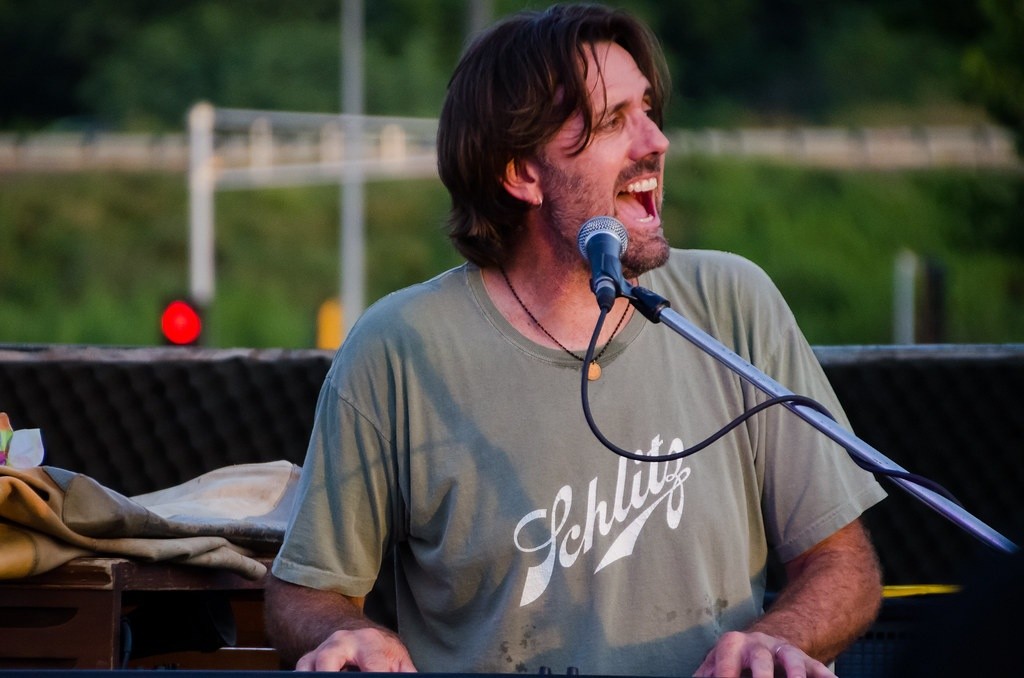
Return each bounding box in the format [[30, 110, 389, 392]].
[[578, 214, 630, 312]]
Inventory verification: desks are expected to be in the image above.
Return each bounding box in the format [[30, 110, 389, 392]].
[[0, 547, 280, 671]]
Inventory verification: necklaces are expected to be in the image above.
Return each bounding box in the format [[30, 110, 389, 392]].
[[496, 263, 633, 381]]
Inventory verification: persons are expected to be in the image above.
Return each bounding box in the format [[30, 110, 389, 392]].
[[264, 4, 892, 677]]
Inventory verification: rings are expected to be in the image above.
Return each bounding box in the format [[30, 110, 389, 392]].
[[774, 641, 801, 661]]
